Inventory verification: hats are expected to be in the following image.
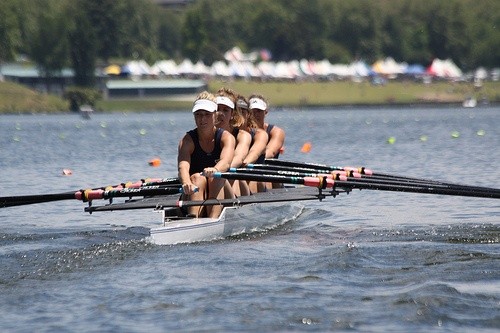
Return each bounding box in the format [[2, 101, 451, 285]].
[[234, 99, 249, 109], [213, 96, 234, 109], [192, 99, 218, 114], [247, 98, 267, 112]]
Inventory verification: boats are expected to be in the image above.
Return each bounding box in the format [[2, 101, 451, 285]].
[[105, 78, 208, 97], [144, 184, 307, 246]]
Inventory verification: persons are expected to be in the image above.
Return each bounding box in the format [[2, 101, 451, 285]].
[[175, 86, 283, 220]]
